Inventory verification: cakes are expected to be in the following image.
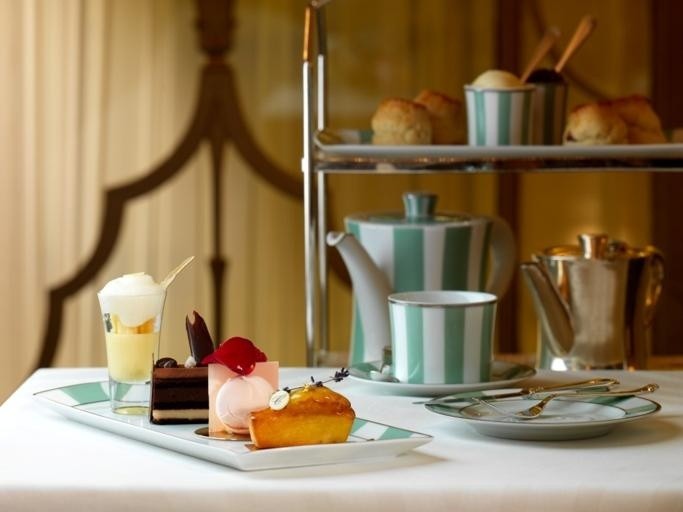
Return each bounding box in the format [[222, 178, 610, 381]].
[[247, 366, 356, 448], [200, 336, 279, 433], [150, 310, 215, 425]]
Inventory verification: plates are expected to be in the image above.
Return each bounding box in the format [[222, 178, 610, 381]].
[[33, 379, 435, 469], [427, 387, 662, 442], [341, 360, 537, 398]]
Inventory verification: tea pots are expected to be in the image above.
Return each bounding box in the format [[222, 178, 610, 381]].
[[324, 193, 517, 376], [521, 231, 666, 371]]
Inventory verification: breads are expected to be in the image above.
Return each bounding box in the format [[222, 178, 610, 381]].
[[562, 97, 668, 145], [369, 88, 463, 144]]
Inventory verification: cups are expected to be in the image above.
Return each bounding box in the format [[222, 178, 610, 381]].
[[98, 290, 168, 415], [465, 85, 569, 147], [387, 290, 500, 383]]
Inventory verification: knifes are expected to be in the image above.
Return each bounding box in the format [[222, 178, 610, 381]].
[[411, 377, 622, 409]]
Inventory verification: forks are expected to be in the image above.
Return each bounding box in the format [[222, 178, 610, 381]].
[[473, 384, 658, 421]]
